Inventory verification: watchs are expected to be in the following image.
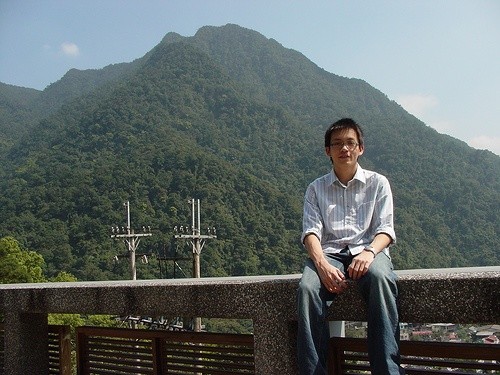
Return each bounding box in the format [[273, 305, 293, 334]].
[[364, 245, 377, 258]]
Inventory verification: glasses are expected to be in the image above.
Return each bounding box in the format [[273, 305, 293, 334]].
[[330, 139, 360, 151]]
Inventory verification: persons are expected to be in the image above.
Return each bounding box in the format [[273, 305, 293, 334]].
[[294, 118, 403, 375]]
